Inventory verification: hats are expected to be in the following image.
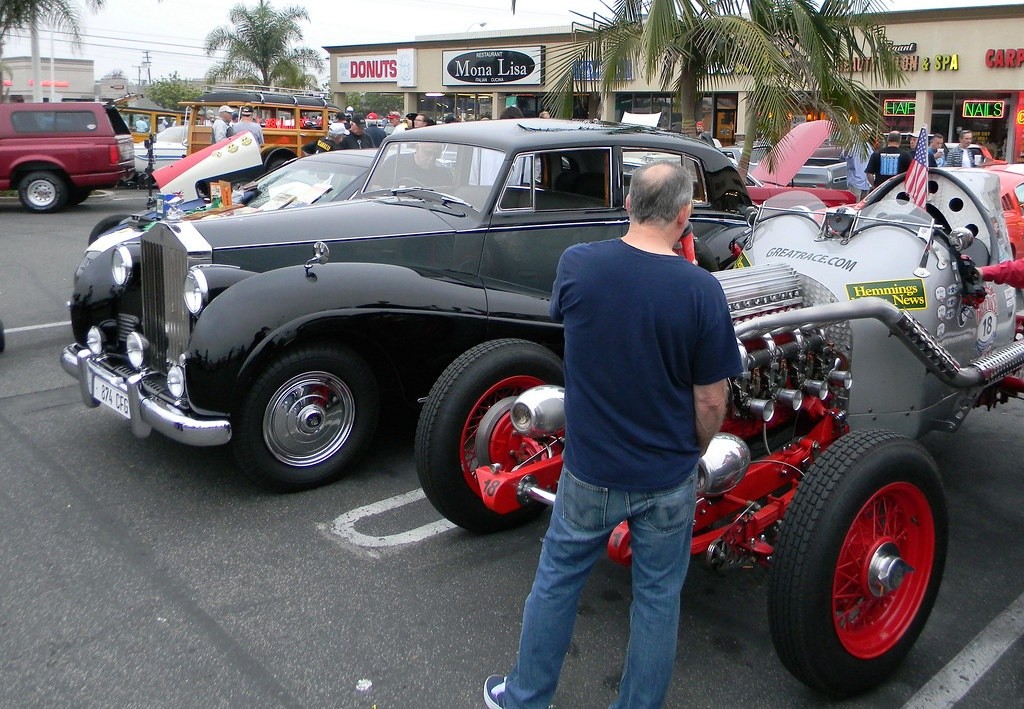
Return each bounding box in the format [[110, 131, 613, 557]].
[[386, 111, 401, 119], [696, 121, 704, 127], [232, 113, 239, 118], [367, 112, 378, 119], [907, 131, 919, 137], [219, 105, 235, 112], [206, 110, 214, 118], [443, 115, 456, 122], [329, 122, 350, 135], [352, 115, 366, 128], [242, 107, 254, 115]]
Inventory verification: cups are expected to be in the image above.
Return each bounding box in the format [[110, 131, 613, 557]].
[[671, 217, 694, 264], [974, 155, 982, 165], [937, 148, 944, 159]]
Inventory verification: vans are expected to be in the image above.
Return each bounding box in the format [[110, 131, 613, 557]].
[[177, 86, 340, 190]]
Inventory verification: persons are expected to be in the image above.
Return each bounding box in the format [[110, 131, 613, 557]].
[[928, 134, 946, 167], [863, 130, 912, 192], [483, 162, 746, 709], [838, 124, 873, 203], [42, 112, 198, 135], [696, 121, 715, 147], [971, 136, 980, 155], [228, 113, 239, 127], [946, 130, 975, 167], [982, 136, 998, 162], [960, 253, 1024, 291], [301, 106, 552, 161], [200, 110, 214, 125], [231, 104, 265, 187], [906, 132, 938, 167], [370, 142, 453, 191], [210, 105, 234, 145], [469, 105, 542, 189]]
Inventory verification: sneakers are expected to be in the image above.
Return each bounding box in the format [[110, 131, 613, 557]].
[[484, 675, 508, 709]]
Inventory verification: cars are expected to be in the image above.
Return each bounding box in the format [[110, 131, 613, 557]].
[[414, 164, 1024, 700], [60, 118, 754, 498], [133, 126, 189, 186], [88, 146, 380, 250]]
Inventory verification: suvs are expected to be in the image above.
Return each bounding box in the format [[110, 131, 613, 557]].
[[1, 103, 133, 212]]
